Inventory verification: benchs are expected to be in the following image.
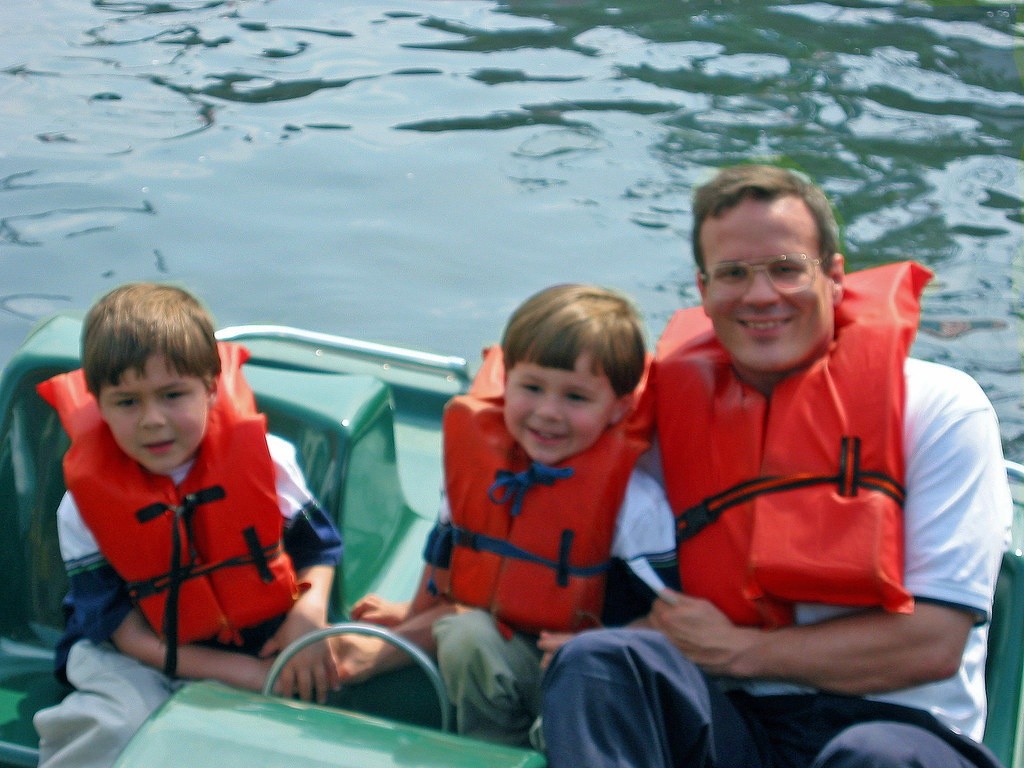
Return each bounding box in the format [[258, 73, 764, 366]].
[[0, 635, 69, 768]]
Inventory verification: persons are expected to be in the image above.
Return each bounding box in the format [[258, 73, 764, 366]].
[[327, 165, 1014, 768], [348, 285, 683, 755], [34, 284, 342, 763]]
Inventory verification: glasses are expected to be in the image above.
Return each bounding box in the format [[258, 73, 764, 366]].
[[701, 254, 829, 295]]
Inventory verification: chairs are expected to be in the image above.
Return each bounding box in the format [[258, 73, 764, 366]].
[[983, 550, 1024, 768]]
